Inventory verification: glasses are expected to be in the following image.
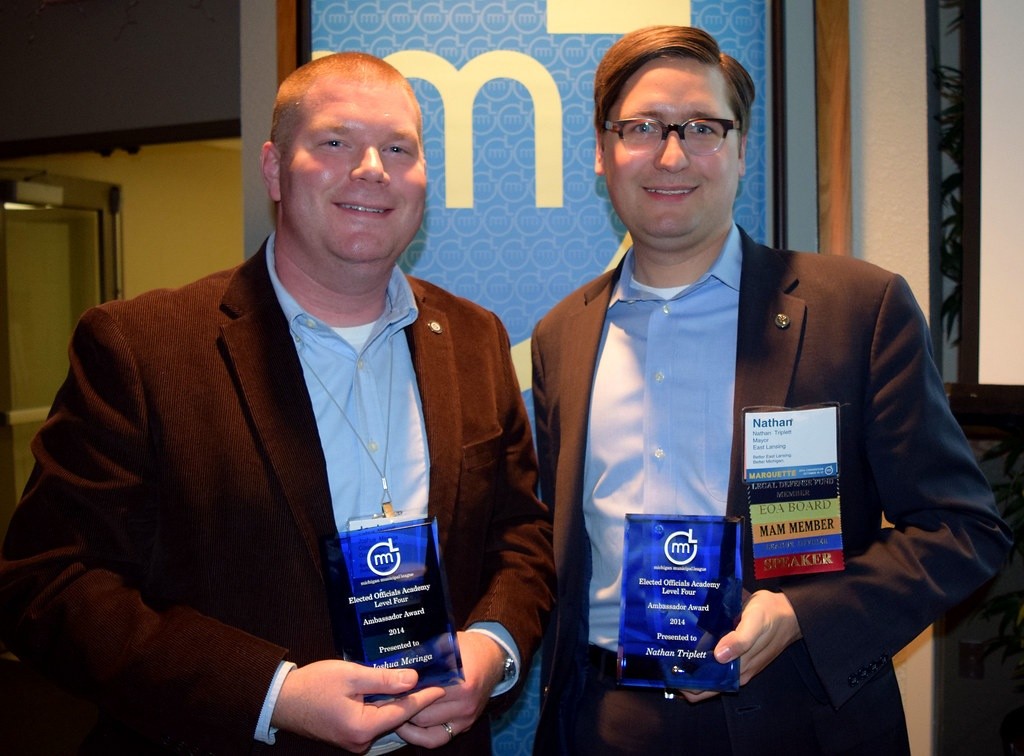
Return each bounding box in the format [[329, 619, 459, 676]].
[[603, 117, 740, 155]]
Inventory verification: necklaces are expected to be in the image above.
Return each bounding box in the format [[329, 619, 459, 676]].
[[279, 323, 397, 521]]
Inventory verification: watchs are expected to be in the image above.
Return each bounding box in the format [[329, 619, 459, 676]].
[[503, 652, 517, 681]]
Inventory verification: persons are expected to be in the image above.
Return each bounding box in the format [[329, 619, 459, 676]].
[[1, 52, 559, 756], [527, 20, 1006, 754]]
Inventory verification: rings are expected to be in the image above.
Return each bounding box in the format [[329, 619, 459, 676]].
[[440, 721, 455, 740]]
